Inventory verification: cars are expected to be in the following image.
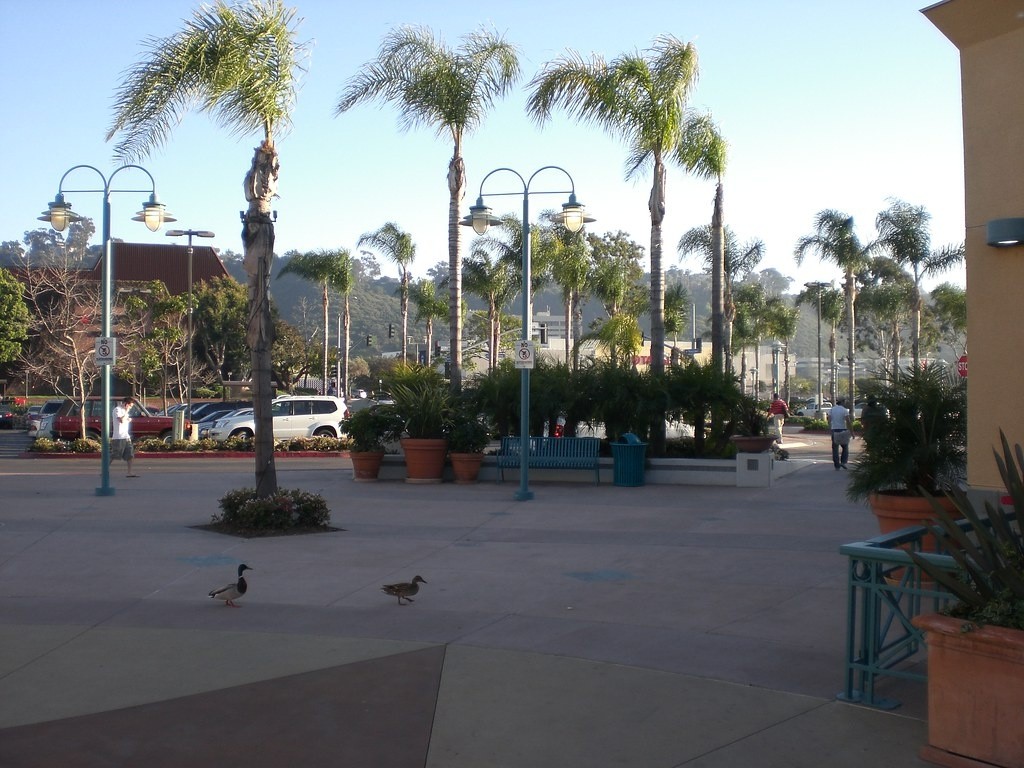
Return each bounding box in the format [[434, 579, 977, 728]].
[[156, 400, 282, 439], [24, 400, 63, 440], [796, 402, 833, 419], [374, 392, 395, 404], [0, 409, 14, 430], [846, 401, 890, 419]]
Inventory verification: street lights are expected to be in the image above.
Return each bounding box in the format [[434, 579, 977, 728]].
[[164, 227, 216, 434], [770, 338, 786, 398], [804, 281, 833, 419], [36, 165, 180, 500], [749, 366, 760, 402], [458, 165, 587, 499]]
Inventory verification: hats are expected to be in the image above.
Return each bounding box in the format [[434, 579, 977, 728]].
[[836, 396, 848, 404]]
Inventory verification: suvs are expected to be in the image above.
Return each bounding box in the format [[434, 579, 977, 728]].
[[209, 394, 351, 440], [50, 395, 192, 446]]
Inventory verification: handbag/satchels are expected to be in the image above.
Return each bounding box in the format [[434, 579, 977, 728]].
[[834, 430, 851, 445], [782, 402, 790, 419]]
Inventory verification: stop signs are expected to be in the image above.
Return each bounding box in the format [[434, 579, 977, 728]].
[[957, 356, 968, 377]]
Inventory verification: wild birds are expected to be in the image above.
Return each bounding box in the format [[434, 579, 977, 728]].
[[209, 564, 254, 608], [383, 574, 428, 606]]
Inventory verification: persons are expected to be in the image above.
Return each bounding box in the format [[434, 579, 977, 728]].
[[861, 394, 886, 456], [99, 397, 140, 478], [828, 397, 855, 471], [767, 393, 789, 444]]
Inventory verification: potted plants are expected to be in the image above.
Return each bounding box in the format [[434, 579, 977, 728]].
[[446, 417, 489, 481], [384, 379, 455, 479], [729, 393, 779, 452], [843, 359, 967, 581], [338, 408, 405, 479], [897, 427, 1023, 767]]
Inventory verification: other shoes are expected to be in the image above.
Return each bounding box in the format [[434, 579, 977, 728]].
[[835, 466, 841, 470], [841, 462, 848, 469], [777, 440, 783, 444], [125, 473, 142, 478]]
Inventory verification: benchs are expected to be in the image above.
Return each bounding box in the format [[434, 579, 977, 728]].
[[496, 437, 601, 487]]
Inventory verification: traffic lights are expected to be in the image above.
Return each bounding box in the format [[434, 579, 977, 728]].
[[367, 334, 373, 346], [389, 323, 395, 339]]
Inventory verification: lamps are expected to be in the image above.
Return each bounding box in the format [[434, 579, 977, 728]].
[[986, 217, 1024, 248]]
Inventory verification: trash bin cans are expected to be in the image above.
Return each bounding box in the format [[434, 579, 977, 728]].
[[609, 433, 649, 487], [187, 424, 199, 441]]
